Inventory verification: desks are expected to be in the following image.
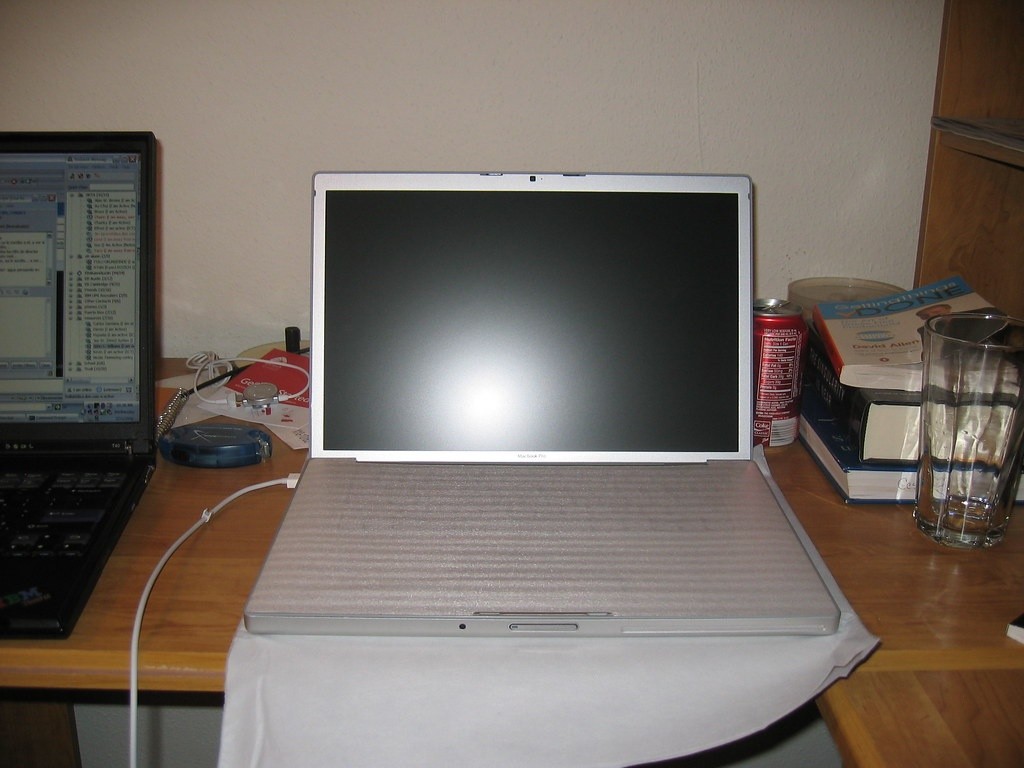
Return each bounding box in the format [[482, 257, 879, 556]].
[[0, 359, 1024, 768]]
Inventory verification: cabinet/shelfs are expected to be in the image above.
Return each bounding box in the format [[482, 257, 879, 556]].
[[916, 0, 1024, 344]]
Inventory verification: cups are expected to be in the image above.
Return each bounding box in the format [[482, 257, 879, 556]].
[[914, 313, 1024, 550], [788, 276, 908, 324]]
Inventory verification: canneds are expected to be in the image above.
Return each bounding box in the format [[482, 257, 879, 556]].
[[752, 298, 808, 448]]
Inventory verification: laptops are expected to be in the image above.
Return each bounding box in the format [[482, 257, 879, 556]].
[[0, 126, 156, 641], [240, 169, 840, 639]]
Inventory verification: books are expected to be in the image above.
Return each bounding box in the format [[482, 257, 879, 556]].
[[152, 386, 190, 444], [798, 275, 1024, 644]]
